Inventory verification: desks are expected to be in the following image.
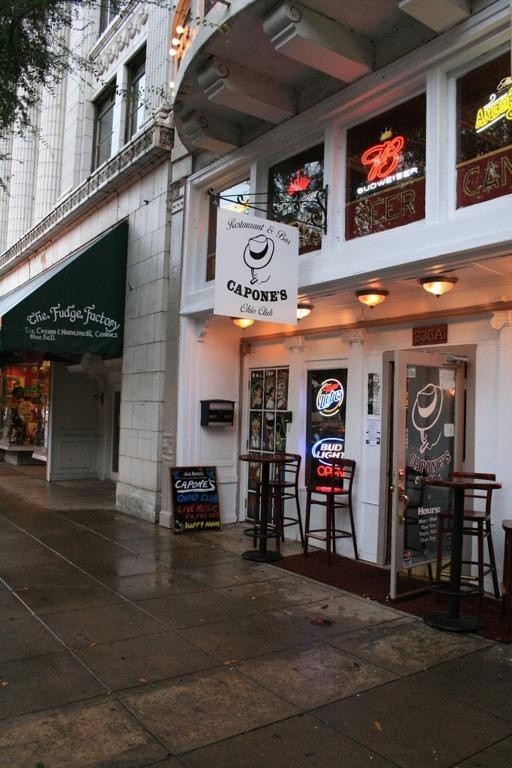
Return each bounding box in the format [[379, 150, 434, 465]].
[[420, 475, 502, 634], [238, 456, 295, 563]]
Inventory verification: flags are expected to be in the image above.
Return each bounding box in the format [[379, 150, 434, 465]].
[[214, 206, 299, 326]]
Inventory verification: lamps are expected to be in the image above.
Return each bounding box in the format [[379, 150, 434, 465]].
[[297, 303, 314, 321], [418, 277, 458, 299], [230, 317, 255, 330], [354, 290, 390, 310]]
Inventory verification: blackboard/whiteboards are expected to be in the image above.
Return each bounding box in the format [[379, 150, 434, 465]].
[[170, 466, 221, 535]]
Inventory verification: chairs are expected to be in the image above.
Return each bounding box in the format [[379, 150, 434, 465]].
[[251, 454, 359, 561], [430, 471, 503, 603]]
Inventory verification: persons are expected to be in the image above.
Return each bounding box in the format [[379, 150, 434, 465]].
[[249, 384, 275, 468]]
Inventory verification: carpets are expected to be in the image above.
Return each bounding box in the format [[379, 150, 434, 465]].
[[269, 548, 511, 646]]
